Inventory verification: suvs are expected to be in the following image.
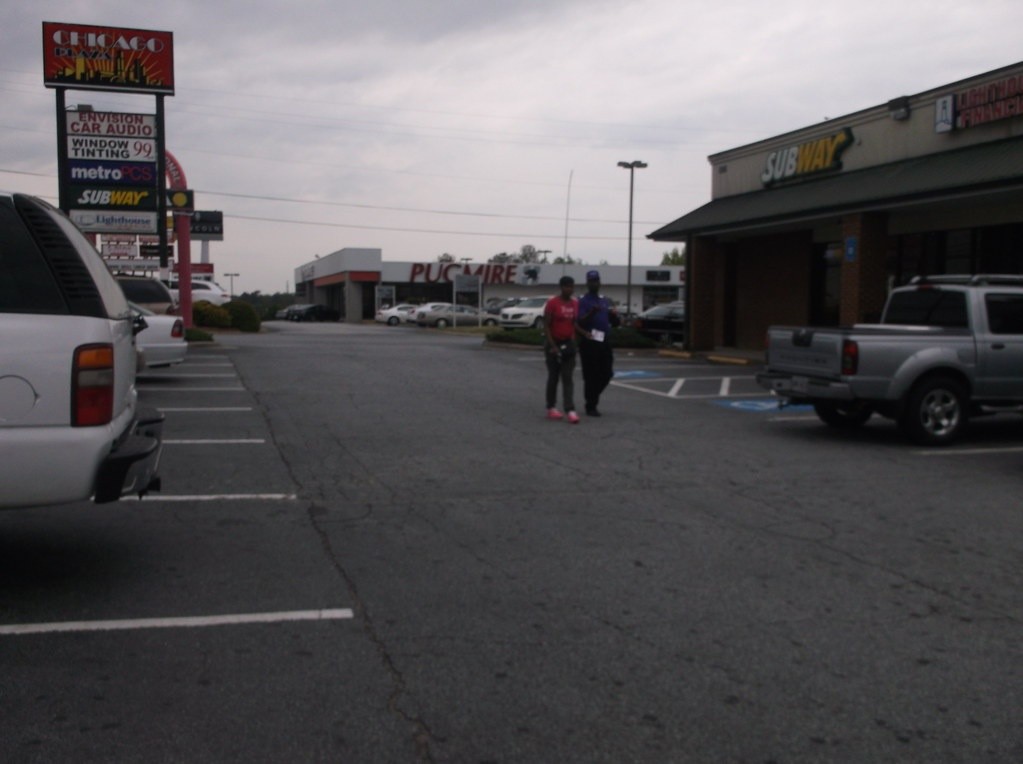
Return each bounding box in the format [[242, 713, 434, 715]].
[[499, 295, 553, 330], [167, 277, 233, 308], [0, 193, 167, 510], [113, 275, 175, 316]]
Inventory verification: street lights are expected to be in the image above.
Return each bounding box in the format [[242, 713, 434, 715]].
[[223, 272, 240, 297], [618, 159, 648, 326], [537, 249, 551, 264]]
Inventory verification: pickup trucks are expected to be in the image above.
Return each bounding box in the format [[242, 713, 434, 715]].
[[754, 270, 1023, 449]]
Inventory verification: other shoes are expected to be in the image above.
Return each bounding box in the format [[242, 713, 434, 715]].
[[586, 405, 601, 416], [568, 413, 579, 423], [548, 412, 563, 421]]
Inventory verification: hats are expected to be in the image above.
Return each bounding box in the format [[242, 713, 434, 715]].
[[587, 271, 599, 280]]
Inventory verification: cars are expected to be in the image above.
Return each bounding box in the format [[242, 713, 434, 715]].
[[125, 296, 188, 373], [415, 302, 500, 329], [374, 303, 420, 327], [407, 302, 451, 328], [600, 295, 638, 326], [633, 301, 684, 341], [275, 303, 342, 323], [482, 296, 527, 315]]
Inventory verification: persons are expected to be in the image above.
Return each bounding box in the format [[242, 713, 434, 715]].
[[544, 276, 593, 424], [577, 271, 621, 416]]
